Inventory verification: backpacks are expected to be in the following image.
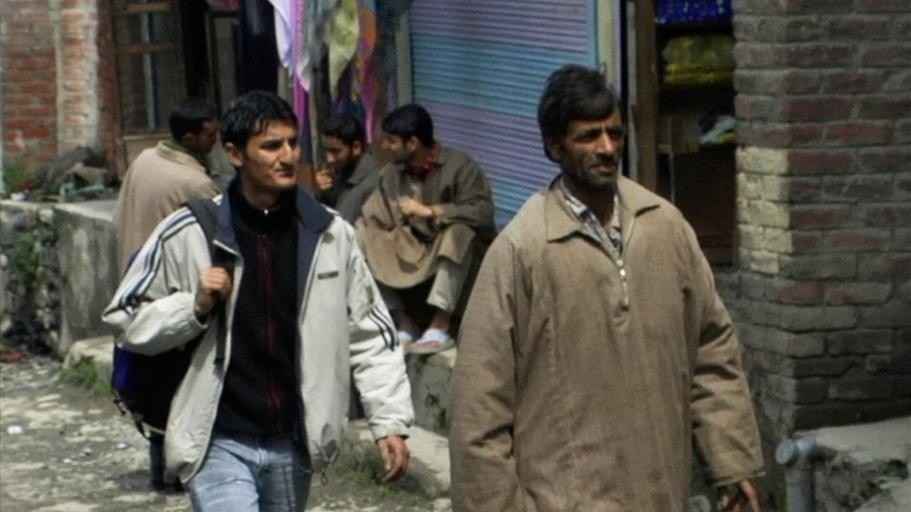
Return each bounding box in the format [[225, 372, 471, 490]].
[[110, 197, 234, 440]]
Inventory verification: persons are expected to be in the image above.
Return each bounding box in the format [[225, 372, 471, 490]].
[[110, 102, 228, 499], [99, 89, 418, 511], [310, 111, 389, 226], [350, 101, 498, 360], [444, 62, 770, 510]]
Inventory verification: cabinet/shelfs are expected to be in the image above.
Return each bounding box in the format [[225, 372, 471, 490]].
[[618, 1, 734, 266]]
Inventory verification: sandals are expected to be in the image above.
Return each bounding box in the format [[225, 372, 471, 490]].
[[394, 325, 455, 355]]
